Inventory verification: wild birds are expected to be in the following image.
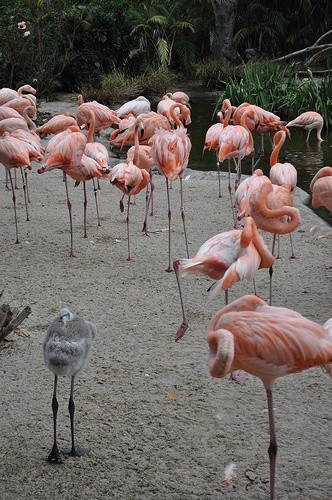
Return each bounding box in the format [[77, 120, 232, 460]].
[[42, 308, 97, 465], [0, 84, 332, 341], [207, 294, 332, 500]]
